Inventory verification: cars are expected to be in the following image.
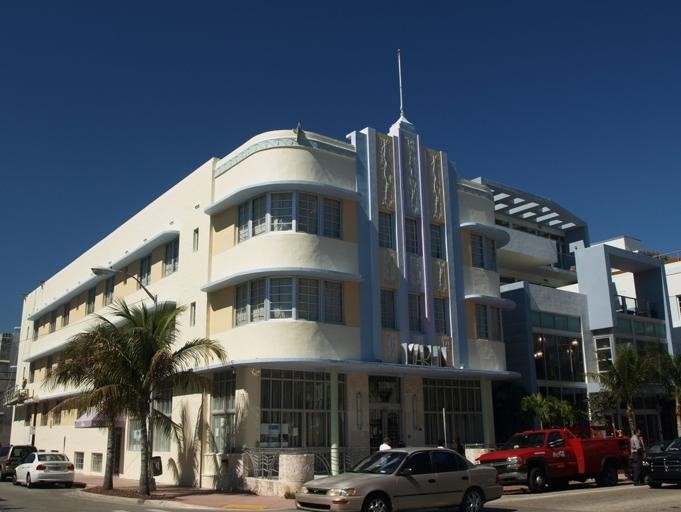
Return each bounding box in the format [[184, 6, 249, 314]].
[[13, 450, 76, 488], [294, 446, 503, 511], [639, 438, 680, 489]]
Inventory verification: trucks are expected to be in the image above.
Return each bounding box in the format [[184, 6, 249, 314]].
[[474, 426, 631, 493]]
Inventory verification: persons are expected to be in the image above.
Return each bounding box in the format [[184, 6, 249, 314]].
[[630, 427, 647, 486], [378, 435, 393, 473], [437, 439, 449, 448]]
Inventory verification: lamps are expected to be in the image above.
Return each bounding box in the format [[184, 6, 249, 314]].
[[571, 338, 579, 347], [536, 347, 543, 360], [357, 392, 362, 430], [634, 272, 640, 280], [412, 394, 417, 431]]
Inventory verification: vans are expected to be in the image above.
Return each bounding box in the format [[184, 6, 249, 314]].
[[1, 444, 38, 482]]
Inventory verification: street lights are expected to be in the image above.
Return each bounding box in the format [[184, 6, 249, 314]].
[[91, 265, 158, 490], [32, 394, 41, 445]]
